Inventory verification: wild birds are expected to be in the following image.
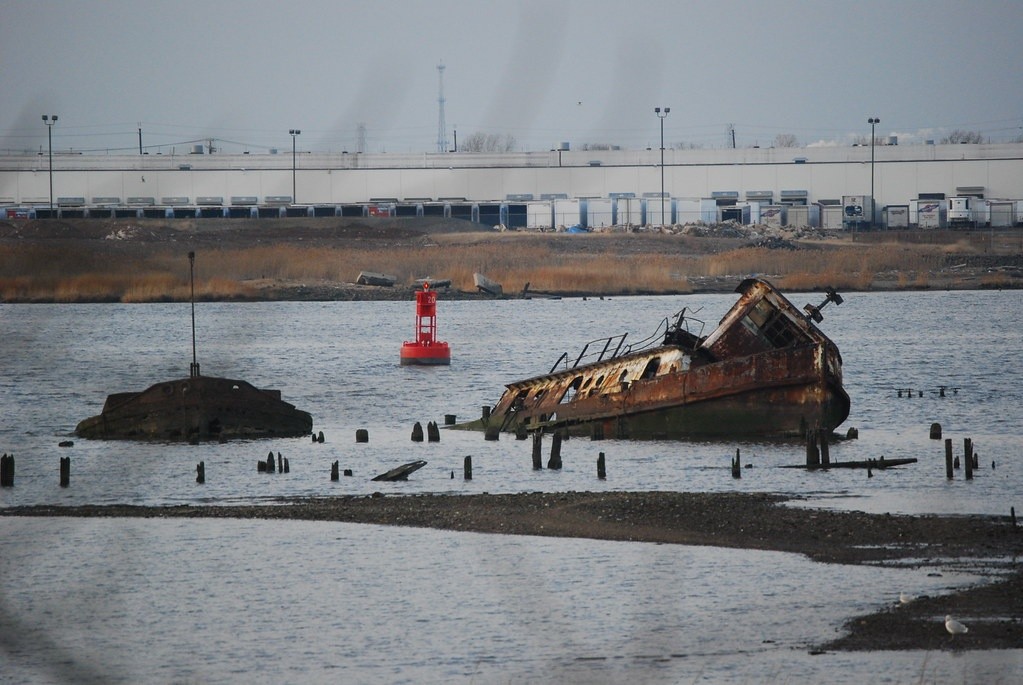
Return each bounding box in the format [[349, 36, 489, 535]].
[[945, 614, 968, 640], [900, 590, 916, 604]]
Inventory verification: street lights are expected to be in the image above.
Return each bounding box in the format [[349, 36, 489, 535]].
[[42, 115, 59, 218], [655, 108, 671, 225], [867, 117, 881, 227], [289, 129, 302, 205]]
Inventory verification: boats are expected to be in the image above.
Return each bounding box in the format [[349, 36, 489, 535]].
[[482, 275, 853, 445], [72, 250, 315, 443]]
